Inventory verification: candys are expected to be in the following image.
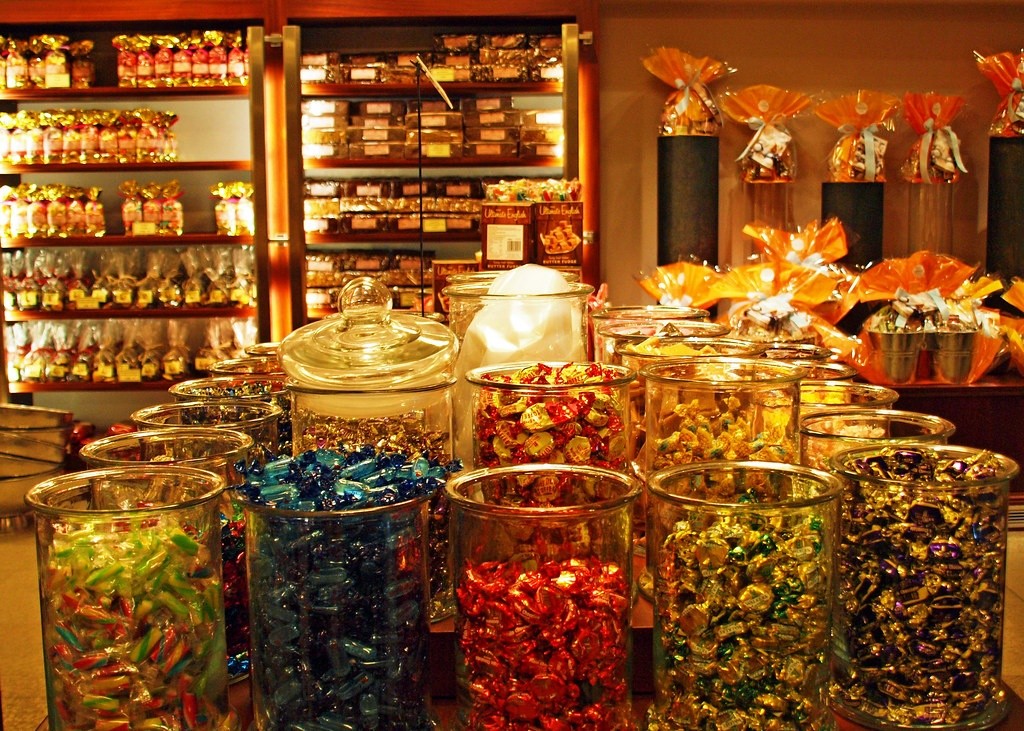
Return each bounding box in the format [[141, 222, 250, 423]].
[[661, 91, 959, 183], [41, 313, 1010, 730]]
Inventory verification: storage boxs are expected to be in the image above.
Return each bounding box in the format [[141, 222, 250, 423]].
[[464, 140, 516, 157], [460, 96, 513, 111], [301, 99, 348, 160], [358, 101, 405, 115], [520, 110, 564, 126], [462, 111, 520, 126], [404, 112, 463, 130], [406, 97, 459, 112], [465, 126, 520, 142], [430, 259, 478, 315], [519, 141, 563, 158], [405, 128, 464, 144], [347, 126, 407, 142], [348, 143, 406, 159], [521, 125, 564, 141], [348, 115, 405, 127], [404, 144, 463, 158], [535, 202, 582, 266], [481, 200, 535, 271]]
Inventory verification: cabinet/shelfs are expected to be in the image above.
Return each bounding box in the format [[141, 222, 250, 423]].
[[282, 23, 580, 333], [0, 26, 271, 407], [853, 369, 1024, 531]]
